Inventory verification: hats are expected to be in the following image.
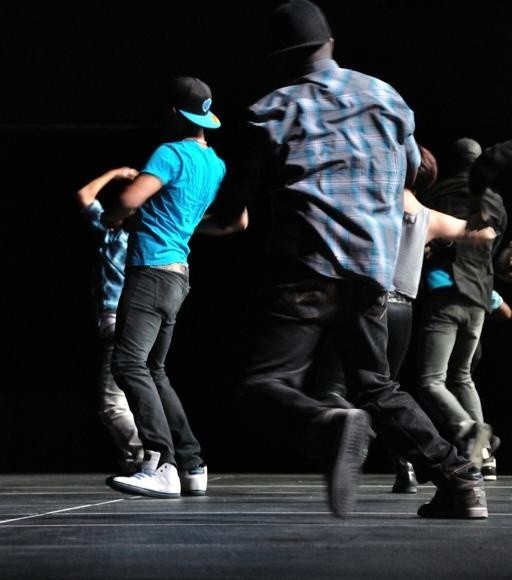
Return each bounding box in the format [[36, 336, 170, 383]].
[[448, 135, 485, 166], [166, 75, 223, 131], [262, 0, 332, 61]]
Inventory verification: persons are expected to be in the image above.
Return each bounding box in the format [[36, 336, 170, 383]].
[[213, 1, 491, 521], [95, 70, 229, 498], [414, 134, 512, 473], [74, 331, 98, 335], [77, 166, 251, 493], [315, 141, 501, 495], [416, 263, 512, 483]]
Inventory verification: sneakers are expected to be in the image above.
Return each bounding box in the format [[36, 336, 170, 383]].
[[415, 472, 490, 521], [480, 458, 499, 481], [390, 461, 419, 495], [458, 421, 500, 464], [104, 451, 186, 504], [322, 408, 373, 521], [179, 465, 209, 498]]
